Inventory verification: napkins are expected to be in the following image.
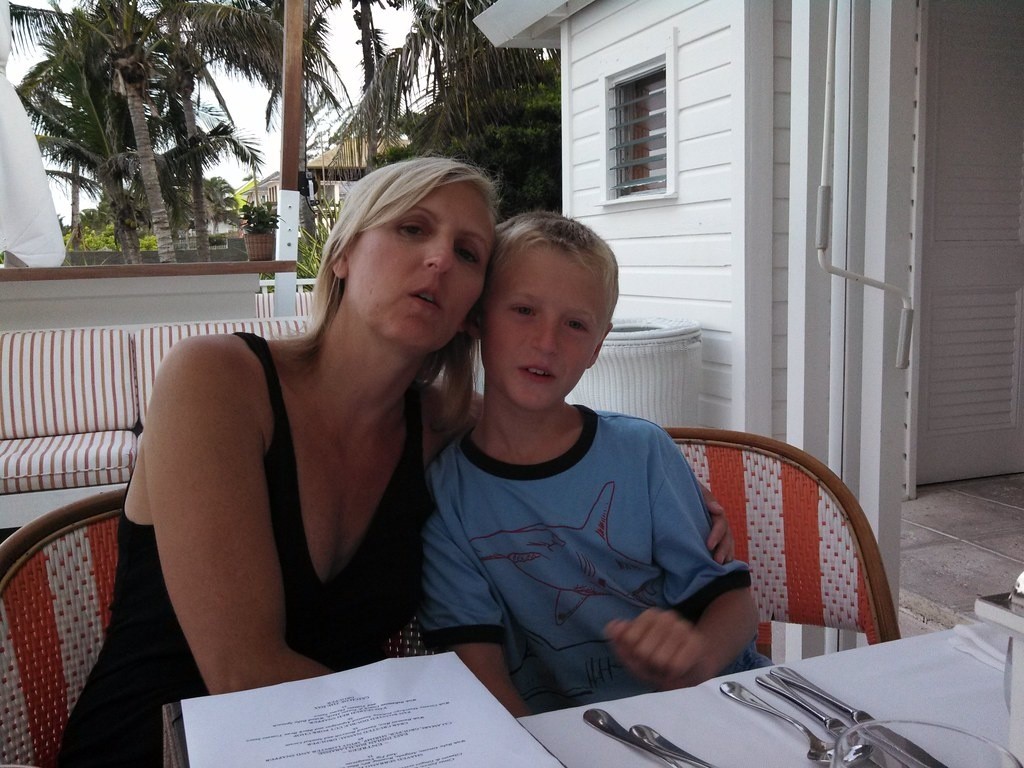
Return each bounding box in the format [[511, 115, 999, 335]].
[[944, 621, 1010, 674]]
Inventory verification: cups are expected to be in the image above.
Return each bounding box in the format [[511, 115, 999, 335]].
[[831, 720, 1023, 768]]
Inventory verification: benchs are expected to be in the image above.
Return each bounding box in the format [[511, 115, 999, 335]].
[[0, 428, 902, 768]]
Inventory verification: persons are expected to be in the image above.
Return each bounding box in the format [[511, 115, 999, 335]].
[[416, 209, 778, 720], [54, 155, 739, 767]]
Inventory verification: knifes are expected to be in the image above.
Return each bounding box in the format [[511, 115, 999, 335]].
[[770, 667, 949, 768], [755, 674, 909, 768]]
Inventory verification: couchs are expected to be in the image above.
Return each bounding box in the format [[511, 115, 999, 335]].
[[0, 318, 315, 533]]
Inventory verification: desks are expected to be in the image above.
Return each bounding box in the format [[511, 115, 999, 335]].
[[516, 619, 1024, 768]]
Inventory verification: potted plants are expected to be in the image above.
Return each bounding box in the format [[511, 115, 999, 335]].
[[237, 198, 286, 261]]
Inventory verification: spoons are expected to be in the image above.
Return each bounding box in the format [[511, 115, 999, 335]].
[[720, 681, 873, 767]]
